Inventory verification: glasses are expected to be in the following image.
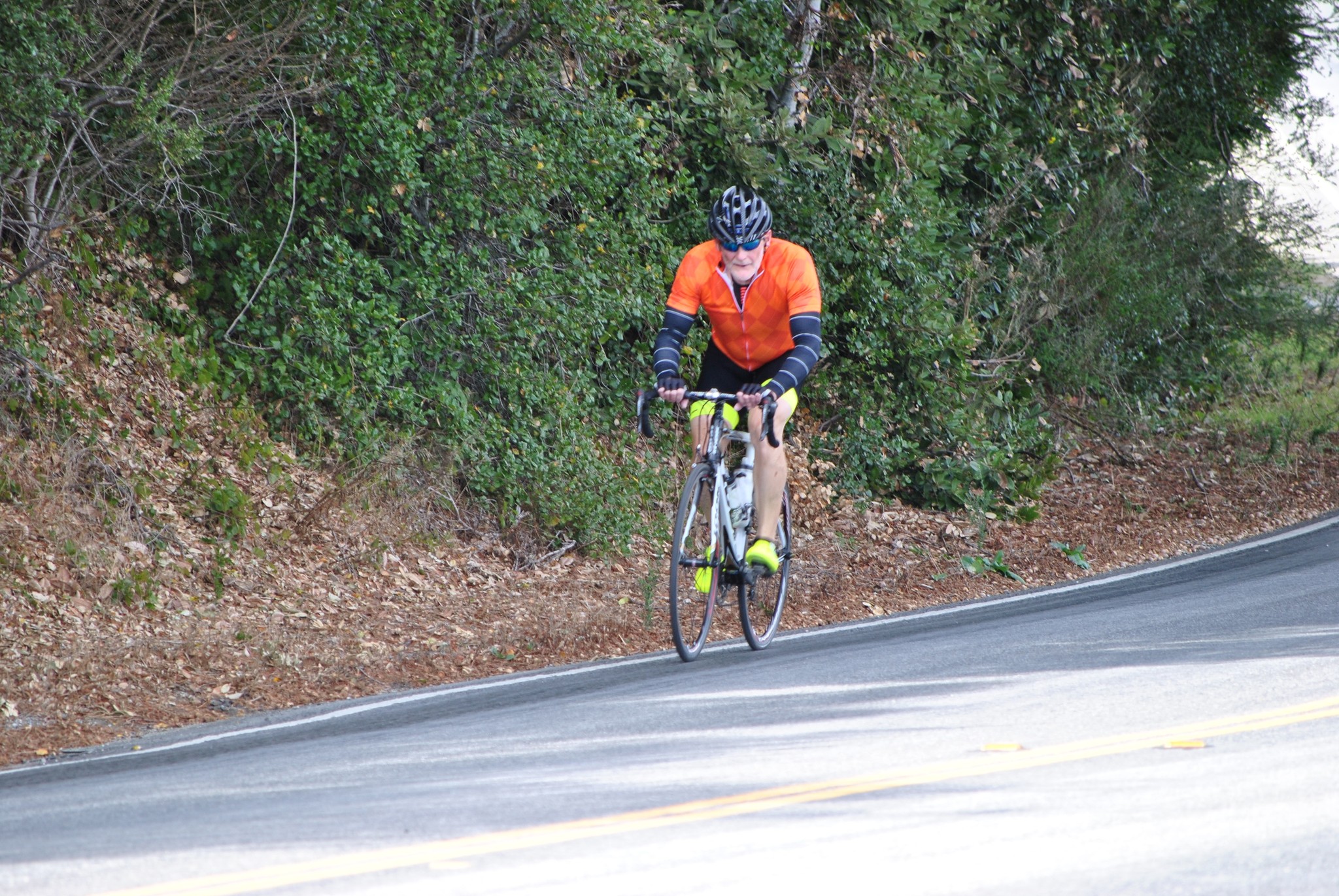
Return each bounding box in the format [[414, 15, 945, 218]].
[[720, 238, 762, 252]]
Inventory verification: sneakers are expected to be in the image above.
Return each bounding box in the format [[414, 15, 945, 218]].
[[745, 539, 779, 575], [694, 546, 725, 593]]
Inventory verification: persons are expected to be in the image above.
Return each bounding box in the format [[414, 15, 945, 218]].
[[653, 186, 821, 592]]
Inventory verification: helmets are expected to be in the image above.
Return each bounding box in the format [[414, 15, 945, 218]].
[[707, 185, 773, 245]]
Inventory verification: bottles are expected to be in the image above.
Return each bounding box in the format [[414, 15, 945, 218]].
[[734, 458, 755, 502], [726, 477, 742, 508]]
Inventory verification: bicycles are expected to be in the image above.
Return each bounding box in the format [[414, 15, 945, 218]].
[[636, 387, 793, 663]]
